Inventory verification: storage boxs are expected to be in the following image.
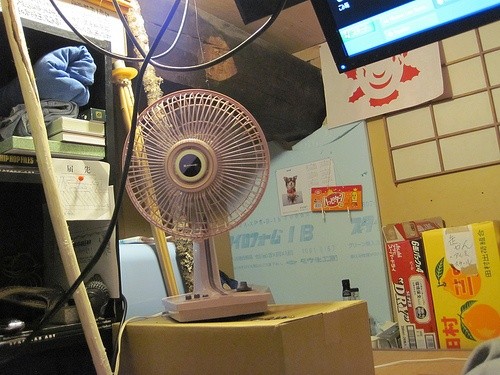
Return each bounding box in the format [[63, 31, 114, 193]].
[[382, 215, 500, 352], [111, 300, 376, 375], [0, 108, 107, 161]]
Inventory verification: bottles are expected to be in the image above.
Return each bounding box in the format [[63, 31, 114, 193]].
[[351, 288, 360, 300], [342, 279, 352, 300]]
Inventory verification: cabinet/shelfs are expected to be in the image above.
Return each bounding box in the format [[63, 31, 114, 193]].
[[0, 10, 124, 375]]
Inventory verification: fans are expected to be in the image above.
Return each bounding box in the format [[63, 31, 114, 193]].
[[121, 89, 271, 323]]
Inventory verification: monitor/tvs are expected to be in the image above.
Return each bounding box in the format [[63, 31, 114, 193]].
[[309, 0, 500, 74]]
